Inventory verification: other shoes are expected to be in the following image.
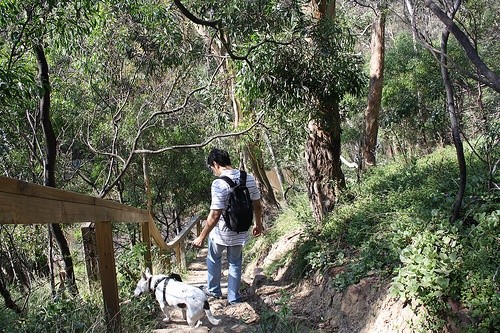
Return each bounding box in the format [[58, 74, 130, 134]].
[[199, 285, 220, 298]]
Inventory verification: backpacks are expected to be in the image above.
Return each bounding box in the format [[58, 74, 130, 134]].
[[213, 169, 254, 232]]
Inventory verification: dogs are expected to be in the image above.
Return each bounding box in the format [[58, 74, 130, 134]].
[[132, 266, 222, 332]]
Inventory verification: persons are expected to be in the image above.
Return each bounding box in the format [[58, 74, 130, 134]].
[[188, 147, 265, 306]]
